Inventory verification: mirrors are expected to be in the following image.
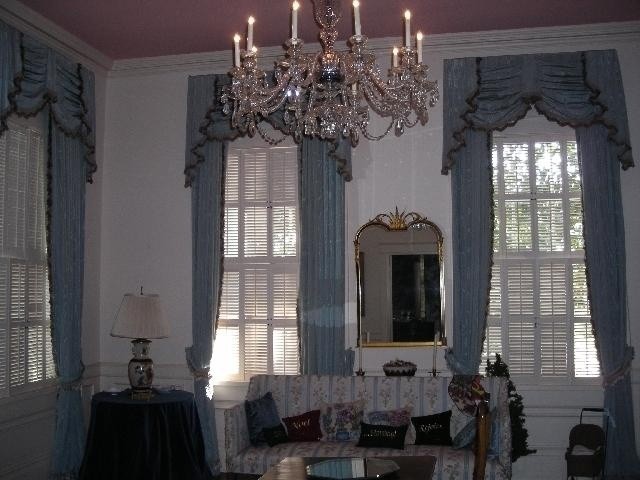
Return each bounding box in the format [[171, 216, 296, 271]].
[[353, 205, 447, 349]]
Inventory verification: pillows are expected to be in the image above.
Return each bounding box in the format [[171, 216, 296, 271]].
[[245, 391, 499, 461]]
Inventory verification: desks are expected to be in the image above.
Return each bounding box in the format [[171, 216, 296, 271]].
[[88, 389, 206, 480], [259, 456, 437, 480]]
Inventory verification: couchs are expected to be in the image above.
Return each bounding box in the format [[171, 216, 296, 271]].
[[224, 373, 511, 480]]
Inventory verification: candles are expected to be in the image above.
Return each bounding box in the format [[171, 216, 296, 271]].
[[433, 334, 437, 370], [437, 331, 440, 341], [359, 334, 362, 370], [367, 331, 370, 343]]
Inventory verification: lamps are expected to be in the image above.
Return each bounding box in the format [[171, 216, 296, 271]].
[[110, 286, 170, 401], [219, 0, 441, 147]]
